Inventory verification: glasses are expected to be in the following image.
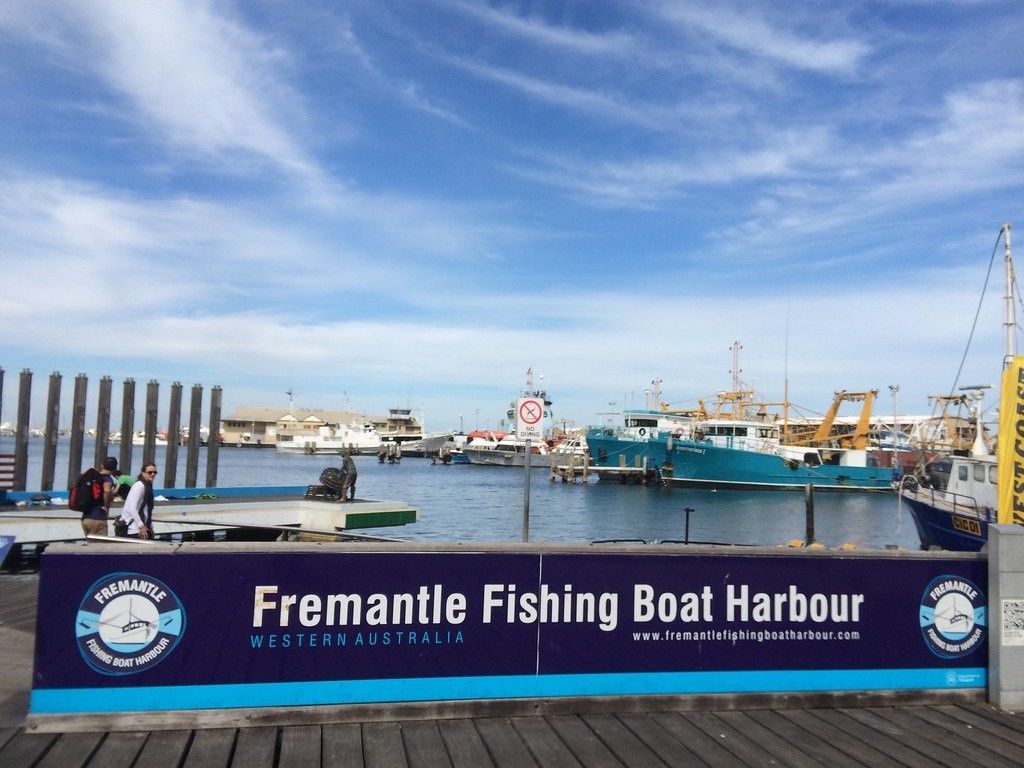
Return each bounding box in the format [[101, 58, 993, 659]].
[[146, 471, 157, 475]]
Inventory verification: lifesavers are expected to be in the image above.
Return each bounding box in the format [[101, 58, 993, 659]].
[[639, 428, 646, 436], [676, 427, 684, 435]]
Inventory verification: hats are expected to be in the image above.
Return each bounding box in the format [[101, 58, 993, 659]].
[[105, 457, 117, 466]]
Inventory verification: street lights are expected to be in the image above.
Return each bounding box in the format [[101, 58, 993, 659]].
[[887, 383, 902, 467]]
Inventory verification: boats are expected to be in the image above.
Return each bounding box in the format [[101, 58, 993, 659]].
[[275, 224, 1024, 550], [88, 425, 212, 446]]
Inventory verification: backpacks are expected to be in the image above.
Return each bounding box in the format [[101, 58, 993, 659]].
[[69, 468, 110, 512]]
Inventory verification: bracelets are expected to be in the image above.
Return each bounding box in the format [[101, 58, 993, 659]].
[[138, 526, 146, 529]]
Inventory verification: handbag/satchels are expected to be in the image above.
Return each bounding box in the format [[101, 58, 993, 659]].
[[112, 517, 128, 537]]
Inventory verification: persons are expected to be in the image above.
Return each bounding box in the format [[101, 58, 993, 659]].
[[335, 447, 356, 503], [81, 457, 157, 539]]
